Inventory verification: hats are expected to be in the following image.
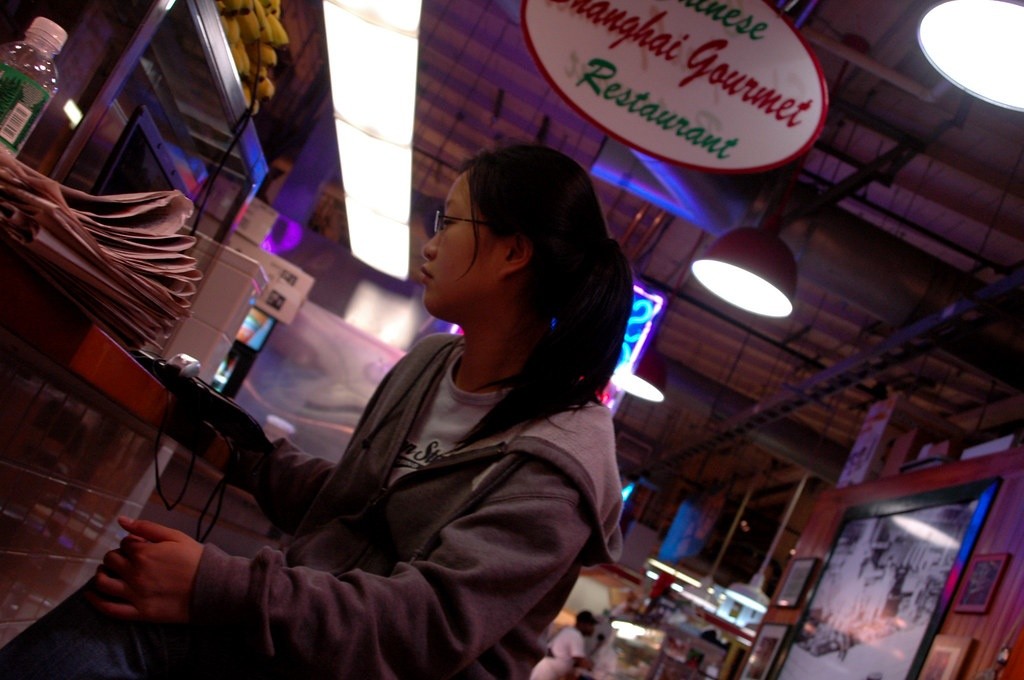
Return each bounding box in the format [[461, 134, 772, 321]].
[[578, 611, 597, 624]]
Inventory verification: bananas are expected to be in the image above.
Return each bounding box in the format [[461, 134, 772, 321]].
[[214, 0, 290, 116]]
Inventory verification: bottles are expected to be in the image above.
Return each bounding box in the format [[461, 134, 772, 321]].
[[0, 17, 68, 158]]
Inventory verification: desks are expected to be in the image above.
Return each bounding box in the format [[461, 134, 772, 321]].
[[0, 226, 243, 492]]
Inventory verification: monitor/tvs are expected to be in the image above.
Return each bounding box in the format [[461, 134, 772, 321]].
[[91, 104, 193, 199]]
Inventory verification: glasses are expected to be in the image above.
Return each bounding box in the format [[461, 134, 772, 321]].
[[434, 210, 497, 233]]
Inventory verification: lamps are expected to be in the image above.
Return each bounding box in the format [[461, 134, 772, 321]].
[[610, 225, 724, 406], [690, 152, 797, 320]]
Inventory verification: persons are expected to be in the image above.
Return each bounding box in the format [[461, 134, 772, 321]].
[[0, 143, 634, 680], [818, 550, 949, 664], [529, 610, 599, 680]]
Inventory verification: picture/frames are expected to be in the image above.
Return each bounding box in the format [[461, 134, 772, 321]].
[[732, 474, 1013, 680]]
